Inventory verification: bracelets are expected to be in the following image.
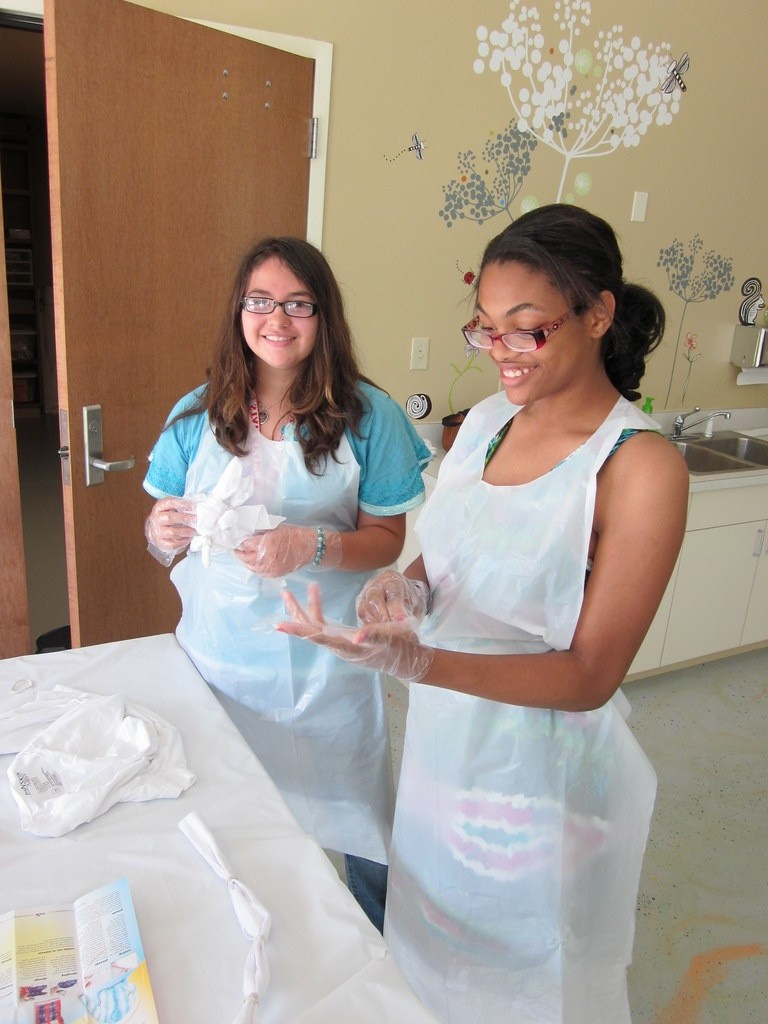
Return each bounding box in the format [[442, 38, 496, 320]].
[[312, 527, 325, 566]]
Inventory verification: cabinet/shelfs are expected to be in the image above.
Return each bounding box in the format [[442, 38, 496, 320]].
[[0, 118, 48, 420], [623, 486, 768, 686]]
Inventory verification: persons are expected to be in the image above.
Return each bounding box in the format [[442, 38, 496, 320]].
[[276, 203, 687, 1024], [142, 239, 431, 937]]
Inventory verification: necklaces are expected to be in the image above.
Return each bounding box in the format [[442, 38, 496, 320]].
[[256, 396, 288, 424]]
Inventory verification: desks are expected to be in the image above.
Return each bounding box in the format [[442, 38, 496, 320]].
[[0, 633, 438, 1024]]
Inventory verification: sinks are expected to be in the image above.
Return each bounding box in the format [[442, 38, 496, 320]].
[[700, 427, 768, 473], [666, 433, 752, 481]]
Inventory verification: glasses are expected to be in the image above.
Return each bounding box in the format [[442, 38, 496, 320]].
[[461, 294, 594, 353], [240, 296, 319, 318]]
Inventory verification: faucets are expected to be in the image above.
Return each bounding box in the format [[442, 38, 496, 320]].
[[672, 406, 732, 440]]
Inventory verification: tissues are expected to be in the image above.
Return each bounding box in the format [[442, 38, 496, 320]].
[[730, 321, 767, 387]]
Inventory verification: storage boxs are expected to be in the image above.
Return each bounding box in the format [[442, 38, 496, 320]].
[[10, 324, 38, 361]]
